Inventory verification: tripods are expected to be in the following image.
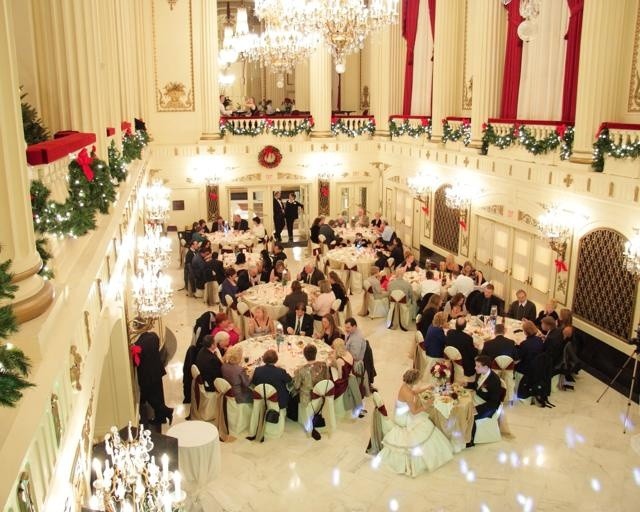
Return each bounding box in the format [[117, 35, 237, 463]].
[[596, 351, 639, 435]]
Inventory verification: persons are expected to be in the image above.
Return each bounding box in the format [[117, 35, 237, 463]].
[[220, 94, 295, 116], [274, 191, 284, 242], [284, 193, 304, 241], [132, 317, 174, 425], [184, 210, 579, 475]]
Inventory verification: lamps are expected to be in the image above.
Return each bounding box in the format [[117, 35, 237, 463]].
[[517, 0, 540, 43], [444, 188, 467, 223], [622, 242, 640, 276], [132, 184, 173, 349], [81, 419, 188, 511], [220, 0, 403, 92], [410, 180, 429, 209]]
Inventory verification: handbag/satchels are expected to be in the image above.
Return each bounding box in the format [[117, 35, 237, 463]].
[[266, 409, 279, 423], [313, 415, 325, 427]]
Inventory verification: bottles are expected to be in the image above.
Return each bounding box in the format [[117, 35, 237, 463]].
[[442, 273, 447, 286], [502, 315, 505, 325], [439, 385, 448, 395]]
